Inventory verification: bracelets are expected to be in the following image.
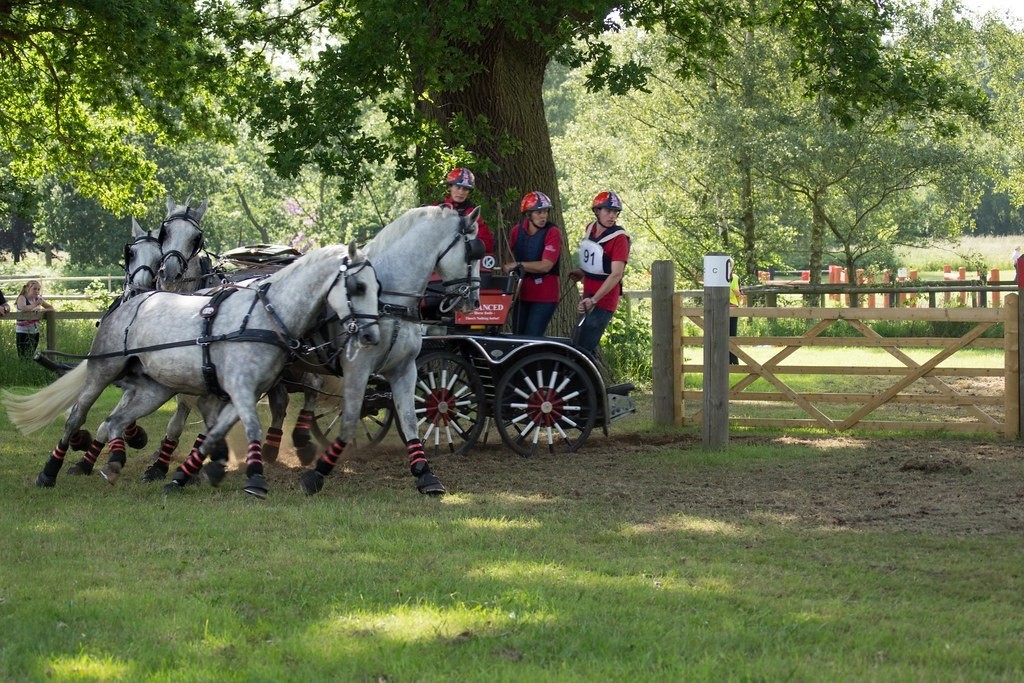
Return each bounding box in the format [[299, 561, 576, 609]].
[[3, 306, 8, 313], [737, 294, 741, 298], [591, 298, 598, 305], [517, 262, 522, 265]]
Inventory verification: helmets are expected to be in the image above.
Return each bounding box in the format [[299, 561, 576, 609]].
[[592, 191, 622, 212], [445, 167, 475, 190], [520, 191, 553, 214]]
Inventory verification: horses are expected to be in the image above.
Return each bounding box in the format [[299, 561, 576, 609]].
[[0, 238, 383, 492], [123, 201, 481, 496]]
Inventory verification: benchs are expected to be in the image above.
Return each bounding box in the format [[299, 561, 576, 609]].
[[429, 268, 522, 336]]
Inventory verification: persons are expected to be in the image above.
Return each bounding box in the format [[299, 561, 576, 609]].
[[888, 271, 895, 307], [423, 167, 494, 313], [729, 257, 746, 365], [1012, 245, 1021, 282], [567, 190, 630, 353], [13, 280, 54, 361], [503, 192, 563, 338], [0, 289, 10, 317]]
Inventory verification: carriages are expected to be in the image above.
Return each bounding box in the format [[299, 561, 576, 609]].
[[123, 193, 640, 464]]
